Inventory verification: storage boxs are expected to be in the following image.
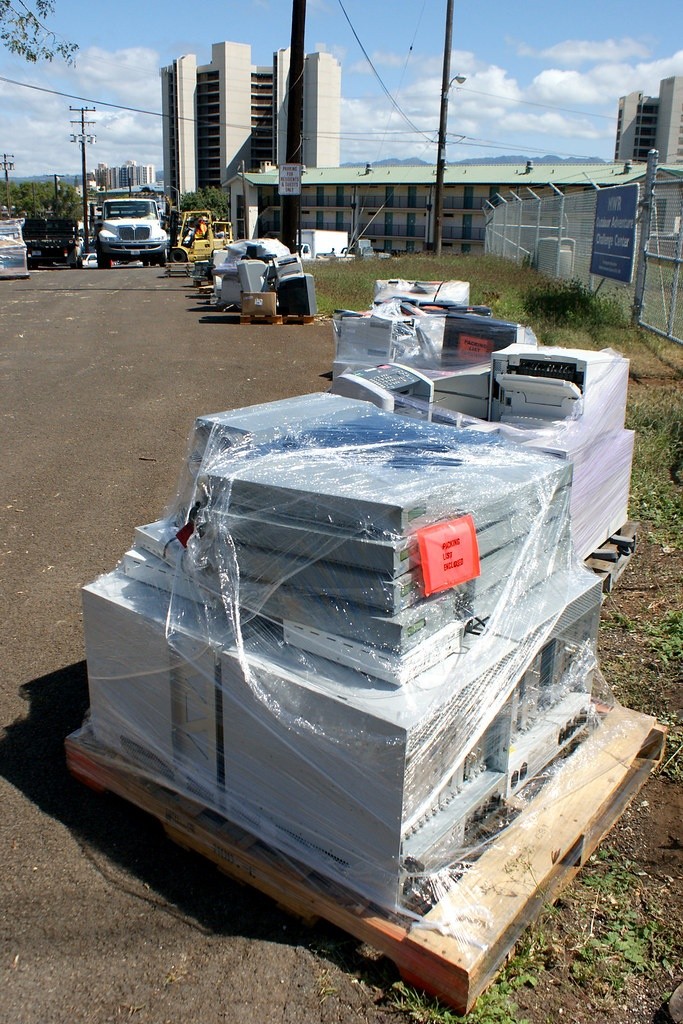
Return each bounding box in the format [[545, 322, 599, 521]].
[[241, 291, 277, 316]]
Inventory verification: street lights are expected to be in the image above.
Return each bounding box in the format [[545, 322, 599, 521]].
[[73, 134, 97, 258], [433, 73, 468, 256], [0, 162, 15, 218], [166, 185, 180, 212]]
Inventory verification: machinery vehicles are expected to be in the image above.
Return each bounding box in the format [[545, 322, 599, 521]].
[[167, 210, 233, 263]]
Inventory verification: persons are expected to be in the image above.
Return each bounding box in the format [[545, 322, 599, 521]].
[[186, 213, 208, 246], [216, 227, 224, 238]]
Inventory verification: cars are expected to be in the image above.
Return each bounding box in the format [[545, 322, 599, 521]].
[[82, 253, 97, 266]]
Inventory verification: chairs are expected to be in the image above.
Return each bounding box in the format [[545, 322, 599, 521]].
[[196, 231, 208, 240]]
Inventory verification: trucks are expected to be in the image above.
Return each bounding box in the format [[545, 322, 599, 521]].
[[21, 218, 83, 269], [96, 199, 169, 270], [295, 229, 350, 258]]
